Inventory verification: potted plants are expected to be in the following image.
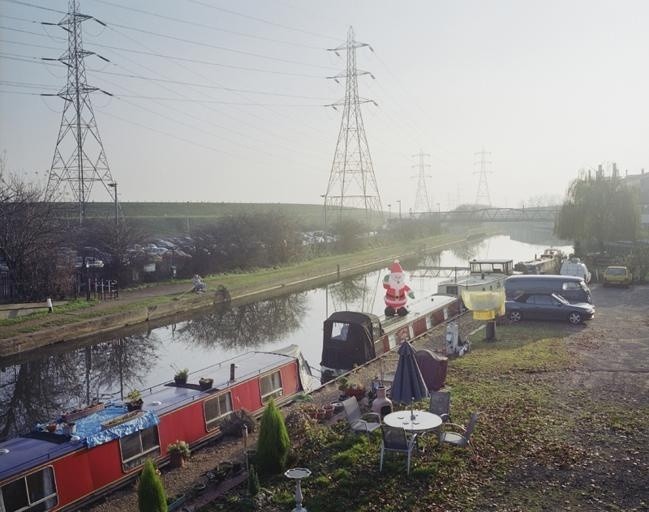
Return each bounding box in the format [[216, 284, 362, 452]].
[[166, 440, 191, 467], [174, 368, 188, 385], [199, 378, 213, 389], [339, 376, 366, 397], [126, 389, 143, 411], [288, 390, 336, 422]]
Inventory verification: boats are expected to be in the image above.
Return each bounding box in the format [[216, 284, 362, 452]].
[[0, 344, 315, 512], [513, 247, 568, 276], [319, 293, 464, 378], [437, 258, 524, 312]]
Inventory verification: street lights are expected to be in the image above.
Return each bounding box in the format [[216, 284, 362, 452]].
[[107, 182, 118, 228]]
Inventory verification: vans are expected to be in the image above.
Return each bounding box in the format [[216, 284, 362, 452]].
[[504, 275, 593, 305]]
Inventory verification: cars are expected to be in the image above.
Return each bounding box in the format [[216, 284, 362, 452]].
[[603, 266, 633, 289], [504, 292, 595, 325]]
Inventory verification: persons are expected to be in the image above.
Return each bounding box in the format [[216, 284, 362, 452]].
[[47, 296, 52, 312], [193, 271, 206, 291]]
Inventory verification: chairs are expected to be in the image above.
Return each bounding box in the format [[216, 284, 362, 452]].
[[342, 391, 477, 476]]
